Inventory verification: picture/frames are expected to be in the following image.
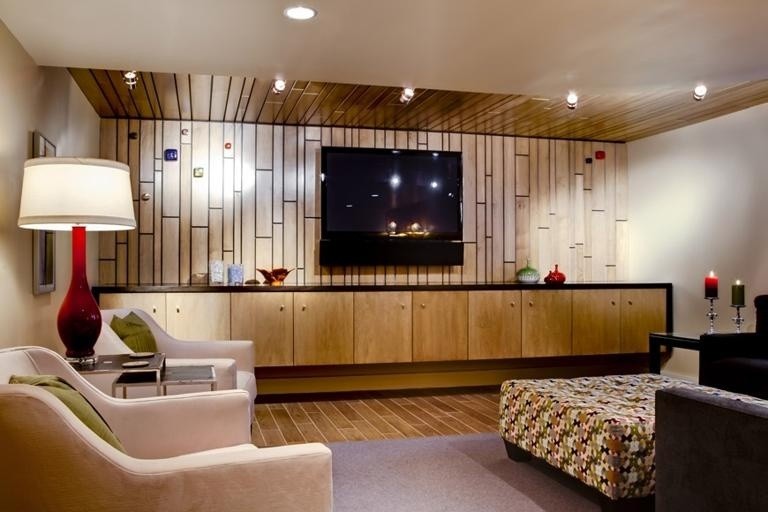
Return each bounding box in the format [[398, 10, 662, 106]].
[[32, 130, 56, 295]]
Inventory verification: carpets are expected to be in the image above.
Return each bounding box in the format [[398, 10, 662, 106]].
[[318, 431, 656, 512]]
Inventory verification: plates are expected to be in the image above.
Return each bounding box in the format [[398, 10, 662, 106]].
[[121, 361, 149, 368], [130, 351, 155, 359]]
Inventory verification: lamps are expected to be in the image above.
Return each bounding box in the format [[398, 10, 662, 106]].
[[124, 70, 138, 90], [399, 88, 415, 104], [17, 156, 137, 366], [271, 80, 286, 96], [694, 84, 706, 101], [567, 94, 577, 109]]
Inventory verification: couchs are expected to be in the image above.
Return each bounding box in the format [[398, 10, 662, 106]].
[[698, 294, 768, 401], [654, 386, 768, 511], [94, 307, 258, 434], [1, 345, 333, 512]]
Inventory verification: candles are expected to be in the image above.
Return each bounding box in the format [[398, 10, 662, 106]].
[[705, 276, 718, 297], [732, 285, 744, 304]]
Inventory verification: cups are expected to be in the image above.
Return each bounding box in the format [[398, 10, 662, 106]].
[[228, 263, 244, 286], [208, 259, 224, 284]]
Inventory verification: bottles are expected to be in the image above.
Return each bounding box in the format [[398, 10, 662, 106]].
[[517, 258, 540, 282], [548, 263, 566, 283]]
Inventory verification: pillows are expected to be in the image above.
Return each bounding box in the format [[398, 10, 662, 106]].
[[109, 311, 158, 352], [9, 373, 129, 456]]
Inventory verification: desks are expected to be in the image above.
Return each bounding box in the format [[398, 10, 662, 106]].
[[111, 364, 217, 398], [61, 351, 165, 401], [648, 331, 701, 376]]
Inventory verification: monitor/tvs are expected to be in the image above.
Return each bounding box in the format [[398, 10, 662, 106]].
[[316, 146, 465, 267]]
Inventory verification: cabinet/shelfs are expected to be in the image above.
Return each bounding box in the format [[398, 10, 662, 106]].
[[230, 292, 353, 367], [571, 288, 666, 356], [469, 289, 571, 360], [99, 292, 230, 342], [354, 290, 469, 365]]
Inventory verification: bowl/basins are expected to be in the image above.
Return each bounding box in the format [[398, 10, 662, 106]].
[[255, 267, 295, 287]]
